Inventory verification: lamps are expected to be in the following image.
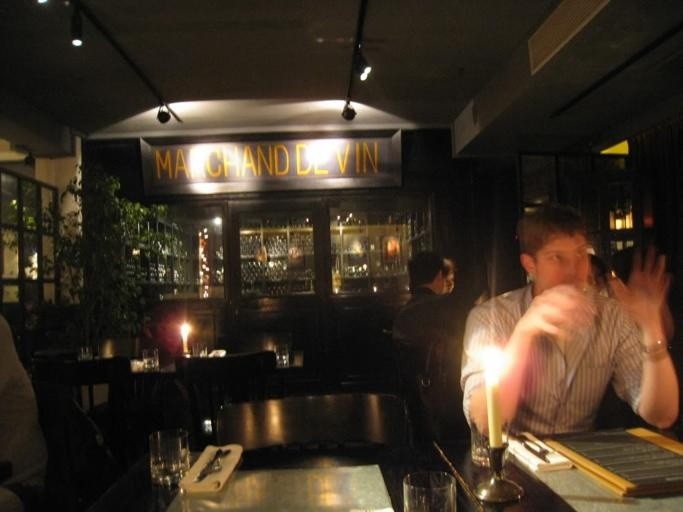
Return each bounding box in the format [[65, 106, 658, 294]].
[[342, 109, 356, 121], [71, 11, 84, 46], [158, 111, 170, 123], [351, 54, 373, 83]]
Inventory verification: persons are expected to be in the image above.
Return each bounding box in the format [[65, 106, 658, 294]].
[[393, 251, 676, 443], [459, 205, 681, 439], [0, 293, 48, 510]]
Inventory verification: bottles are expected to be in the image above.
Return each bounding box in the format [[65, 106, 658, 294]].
[[238, 206, 431, 297], [607, 196, 634, 252]]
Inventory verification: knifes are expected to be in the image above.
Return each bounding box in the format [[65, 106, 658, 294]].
[[194, 448, 222, 484]]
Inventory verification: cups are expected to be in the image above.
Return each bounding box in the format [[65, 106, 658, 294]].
[[470, 416, 510, 468], [191, 341, 208, 357], [272, 344, 290, 368], [402, 471, 458, 512], [143, 348, 160, 369], [149, 427, 190, 488]]
